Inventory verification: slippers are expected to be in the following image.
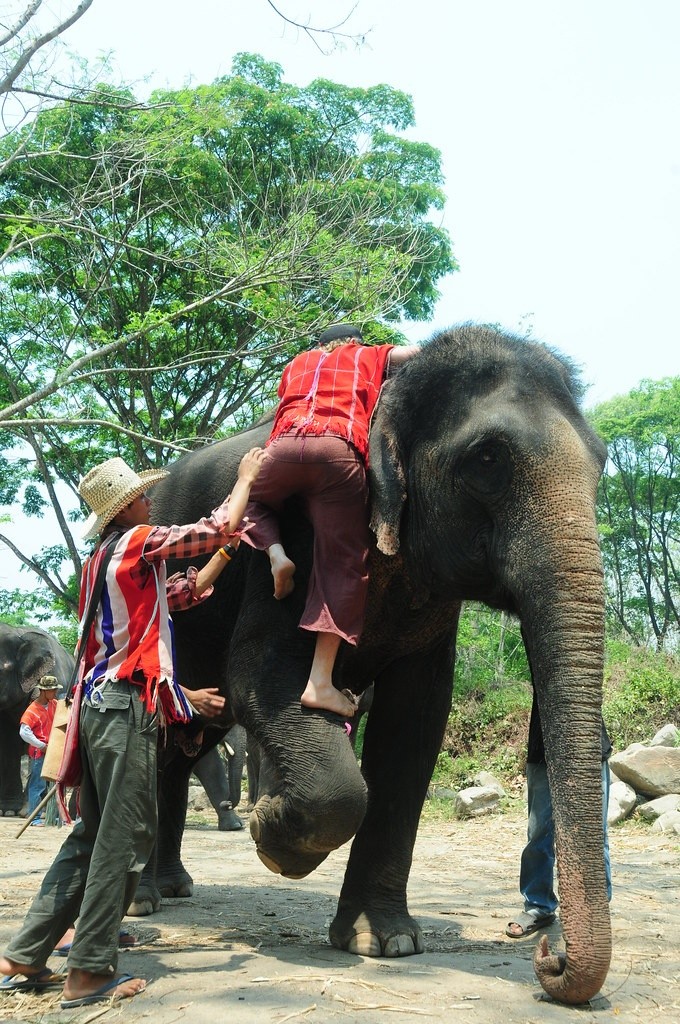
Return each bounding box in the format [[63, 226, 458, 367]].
[[60, 973, 146, 1008], [50, 943, 73, 957], [506, 911, 556, 938], [0, 968, 69, 990], [119, 931, 140, 947]]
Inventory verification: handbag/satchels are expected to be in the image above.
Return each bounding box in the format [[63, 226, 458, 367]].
[[40, 699, 74, 783]]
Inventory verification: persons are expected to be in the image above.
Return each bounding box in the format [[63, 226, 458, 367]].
[[0, 447, 270, 1010], [505, 677, 612, 938], [244, 323, 422, 718]]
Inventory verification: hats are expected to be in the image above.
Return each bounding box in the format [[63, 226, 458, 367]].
[[319, 325, 375, 347], [78, 457, 170, 540]]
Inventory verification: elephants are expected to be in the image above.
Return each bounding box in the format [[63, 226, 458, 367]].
[[0, 621, 83, 829], [87, 320, 616, 1011]]
